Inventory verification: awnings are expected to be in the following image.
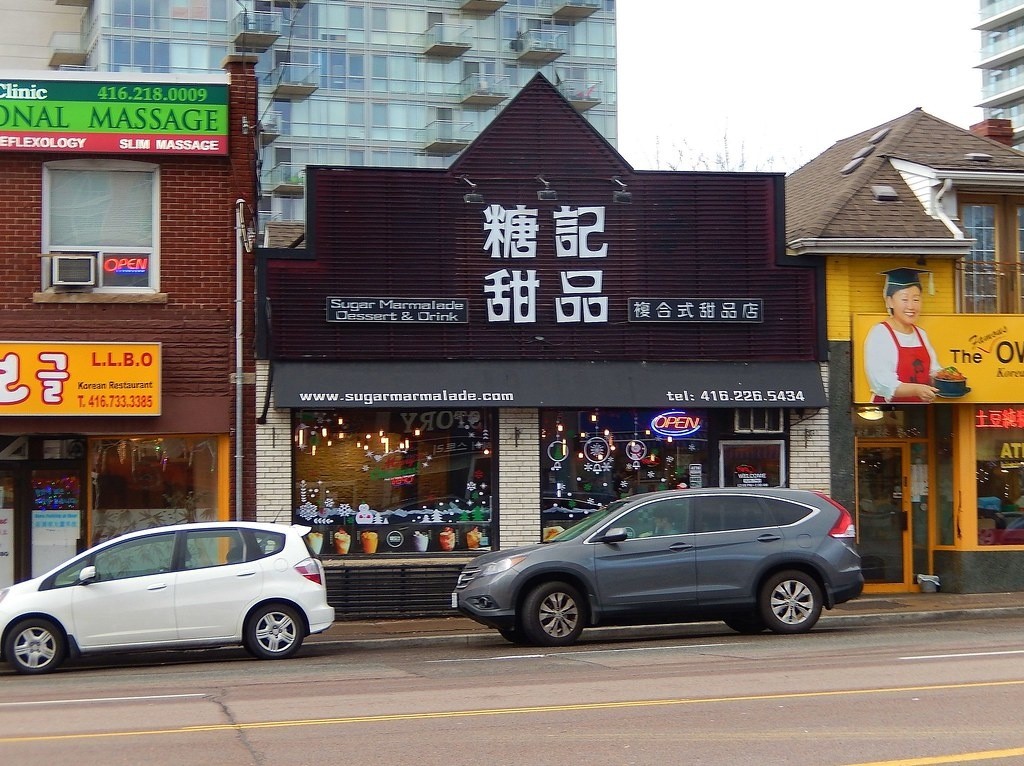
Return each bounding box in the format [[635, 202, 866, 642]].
[[273, 362, 828, 408]]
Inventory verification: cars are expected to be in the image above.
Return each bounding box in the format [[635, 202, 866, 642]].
[[0, 522, 335, 674]]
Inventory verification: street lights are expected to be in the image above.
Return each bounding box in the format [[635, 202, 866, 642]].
[[234, 199, 255, 521]]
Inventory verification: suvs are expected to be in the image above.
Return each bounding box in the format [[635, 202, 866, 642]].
[[450, 487, 865, 646]]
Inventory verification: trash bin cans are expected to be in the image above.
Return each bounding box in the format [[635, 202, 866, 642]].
[[917, 573, 940, 593]]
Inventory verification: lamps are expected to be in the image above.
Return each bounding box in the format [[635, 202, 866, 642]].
[[610, 177, 633, 204], [460, 176, 487, 204], [533, 176, 559, 201]]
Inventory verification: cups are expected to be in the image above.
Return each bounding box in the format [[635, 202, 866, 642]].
[[361, 537, 378, 553], [467, 532, 480, 548], [309, 538, 323, 554], [439, 533, 455, 551], [334, 536, 350, 554], [414, 534, 428, 551]]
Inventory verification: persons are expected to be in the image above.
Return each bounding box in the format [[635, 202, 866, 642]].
[[639, 503, 685, 537], [863, 266, 944, 403]]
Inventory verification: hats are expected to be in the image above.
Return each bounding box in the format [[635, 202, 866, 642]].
[[880, 267, 934, 295]]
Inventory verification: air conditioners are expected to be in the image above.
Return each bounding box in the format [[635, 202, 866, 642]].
[[51, 254, 95, 287]]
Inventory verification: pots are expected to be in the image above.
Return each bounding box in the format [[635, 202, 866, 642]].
[[934, 375, 966, 397]]
